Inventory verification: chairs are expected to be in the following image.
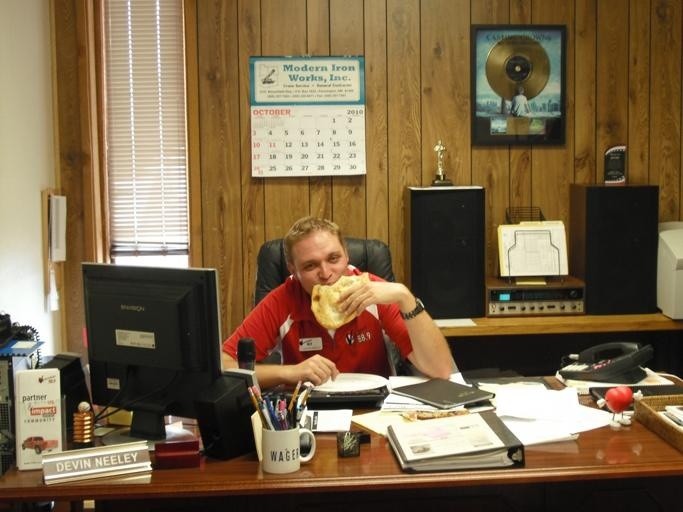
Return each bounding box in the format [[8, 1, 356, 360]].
[[254, 238, 408, 379]]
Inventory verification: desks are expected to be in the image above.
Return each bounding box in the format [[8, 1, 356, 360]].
[[0, 375, 683, 512], [433, 314, 683, 338]]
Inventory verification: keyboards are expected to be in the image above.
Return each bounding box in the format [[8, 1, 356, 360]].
[[263, 386, 388, 410]]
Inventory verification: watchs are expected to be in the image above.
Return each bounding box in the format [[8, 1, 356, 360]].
[[400, 297, 425, 320]]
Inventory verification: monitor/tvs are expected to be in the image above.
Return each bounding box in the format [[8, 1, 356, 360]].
[[80, 262, 222, 456]]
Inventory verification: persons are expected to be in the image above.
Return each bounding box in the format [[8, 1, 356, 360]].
[[510, 86, 530, 117], [222, 217, 452, 388]]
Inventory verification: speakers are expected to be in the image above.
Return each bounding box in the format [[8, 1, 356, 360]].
[[405, 186, 486, 320], [194, 367, 264, 461], [570, 182, 659, 315], [39, 351, 95, 434]]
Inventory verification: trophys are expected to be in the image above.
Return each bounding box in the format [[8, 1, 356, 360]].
[[431, 140, 453, 186]]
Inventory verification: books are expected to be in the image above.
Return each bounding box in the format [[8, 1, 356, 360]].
[[589, 385, 683, 414], [556, 367, 675, 395], [392, 377, 496, 410]]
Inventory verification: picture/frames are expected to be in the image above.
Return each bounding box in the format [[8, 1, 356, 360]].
[[468, 24, 568, 149]]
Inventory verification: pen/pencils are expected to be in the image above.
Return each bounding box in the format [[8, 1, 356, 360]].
[[248, 381, 314, 431]]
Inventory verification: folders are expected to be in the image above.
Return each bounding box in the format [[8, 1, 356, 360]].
[[386, 410, 526, 473]]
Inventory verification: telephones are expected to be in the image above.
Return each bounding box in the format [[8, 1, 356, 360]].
[[559, 342, 654, 385]]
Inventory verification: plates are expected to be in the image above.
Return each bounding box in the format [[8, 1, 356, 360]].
[[303, 371, 390, 393]]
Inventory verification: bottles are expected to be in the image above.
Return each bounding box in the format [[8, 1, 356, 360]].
[[72, 409, 95, 449]]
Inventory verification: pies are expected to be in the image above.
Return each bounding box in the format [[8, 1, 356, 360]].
[[310, 273, 370, 330]]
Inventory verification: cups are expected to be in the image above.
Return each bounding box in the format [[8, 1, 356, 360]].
[[260, 423, 317, 474]]
